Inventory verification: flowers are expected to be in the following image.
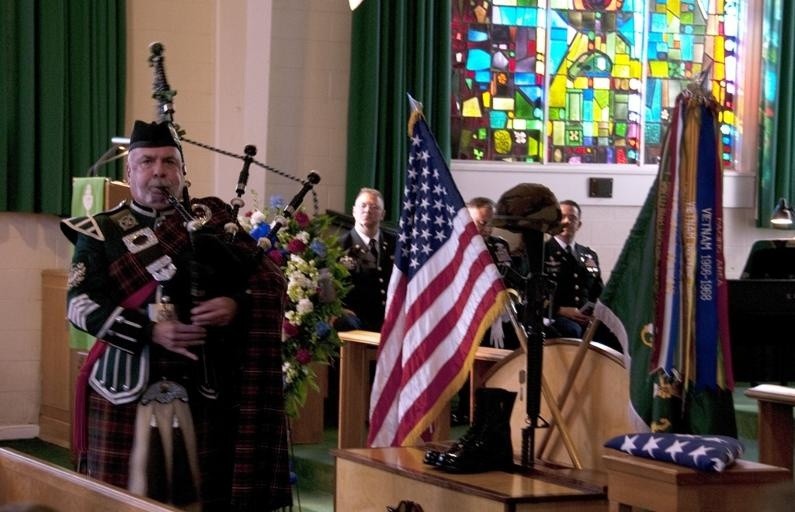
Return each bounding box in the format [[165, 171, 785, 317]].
[[234, 194, 363, 422]]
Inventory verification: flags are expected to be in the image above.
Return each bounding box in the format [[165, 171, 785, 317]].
[[364, 108, 511, 449], [594, 86, 739, 435]]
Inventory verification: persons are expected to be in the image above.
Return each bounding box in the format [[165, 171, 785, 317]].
[[334, 187, 398, 331], [542, 200, 604, 338], [59, 120, 293, 512], [468, 197, 524, 350]]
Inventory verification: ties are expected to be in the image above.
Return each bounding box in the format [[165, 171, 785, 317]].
[[369, 240, 380, 259], [565, 245, 576, 261]]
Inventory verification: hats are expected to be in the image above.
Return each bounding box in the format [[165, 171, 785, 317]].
[[127, 118, 181, 149]]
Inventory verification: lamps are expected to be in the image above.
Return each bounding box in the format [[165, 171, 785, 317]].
[[769, 198, 795, 224]]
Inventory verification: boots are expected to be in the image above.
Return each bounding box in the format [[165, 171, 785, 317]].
[[423, 385, 518, 476]]
